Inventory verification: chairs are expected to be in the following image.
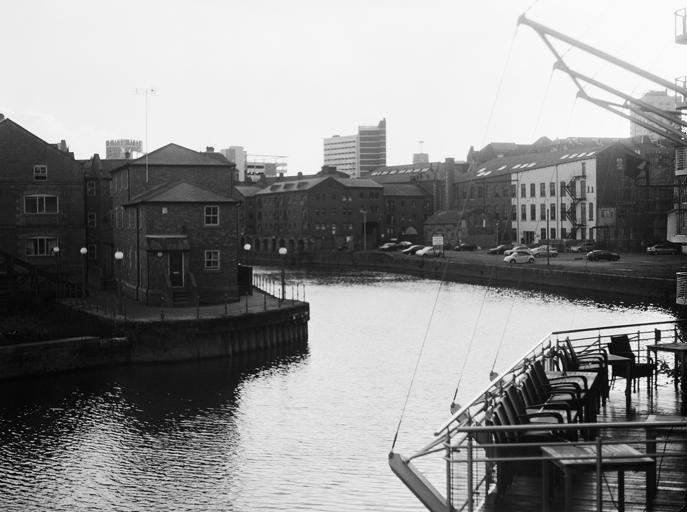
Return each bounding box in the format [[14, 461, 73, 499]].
[[482, 328, 687, 512]]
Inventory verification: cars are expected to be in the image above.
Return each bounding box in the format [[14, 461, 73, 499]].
[[380, 241, 444, 257], [571, 240, 619, 261], [647, 245, 679, 255], [454, 243, 477, 250], [487, 242, 559, 264]]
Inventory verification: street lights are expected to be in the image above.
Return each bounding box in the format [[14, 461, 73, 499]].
[[279, 247, 287, 301], [115, 251, 123, 314], [80, 247, 87, 308], [244, 243, 251, 295], [54, 247, 60, 302]]
